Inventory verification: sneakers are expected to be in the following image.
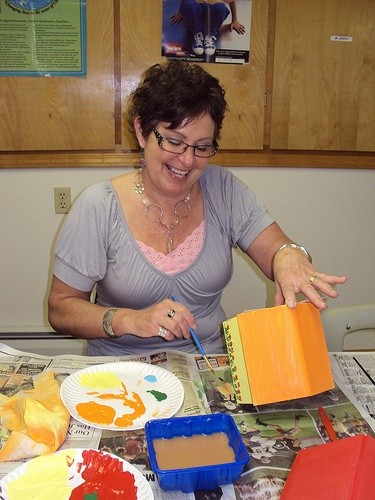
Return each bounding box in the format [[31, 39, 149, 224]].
[[192, 32, 204, 55], [204, 34, 217, 56]]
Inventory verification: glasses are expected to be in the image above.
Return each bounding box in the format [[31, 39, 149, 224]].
[[153, 128, 219, 158]]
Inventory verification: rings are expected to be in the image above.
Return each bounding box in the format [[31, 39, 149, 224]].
[[159, 325, 167, 336], [167, 310, 175, 319], [310, 274, 315, 285]]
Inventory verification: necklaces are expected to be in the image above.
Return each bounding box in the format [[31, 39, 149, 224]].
[[133, 167, 194, 255]]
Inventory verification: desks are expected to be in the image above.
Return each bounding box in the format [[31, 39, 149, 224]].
[[0, 349, 375, 500]]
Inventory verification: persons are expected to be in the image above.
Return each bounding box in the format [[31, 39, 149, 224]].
[[210, 376, 367, 464], [170, 0, 245, 57], [48, 60, 348, 357]]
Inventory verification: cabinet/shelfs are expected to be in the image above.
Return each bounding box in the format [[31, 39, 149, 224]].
[[0, 0, 375, 171]]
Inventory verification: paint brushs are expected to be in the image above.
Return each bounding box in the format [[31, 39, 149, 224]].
[[171, 294, 215, 376]]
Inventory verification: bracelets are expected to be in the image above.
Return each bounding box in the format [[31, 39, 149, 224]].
[[272, 243, 312, 280], [101, 308, 120, 339]]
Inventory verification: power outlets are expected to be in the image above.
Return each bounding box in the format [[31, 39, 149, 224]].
[[54, 188, 71, 214]]
